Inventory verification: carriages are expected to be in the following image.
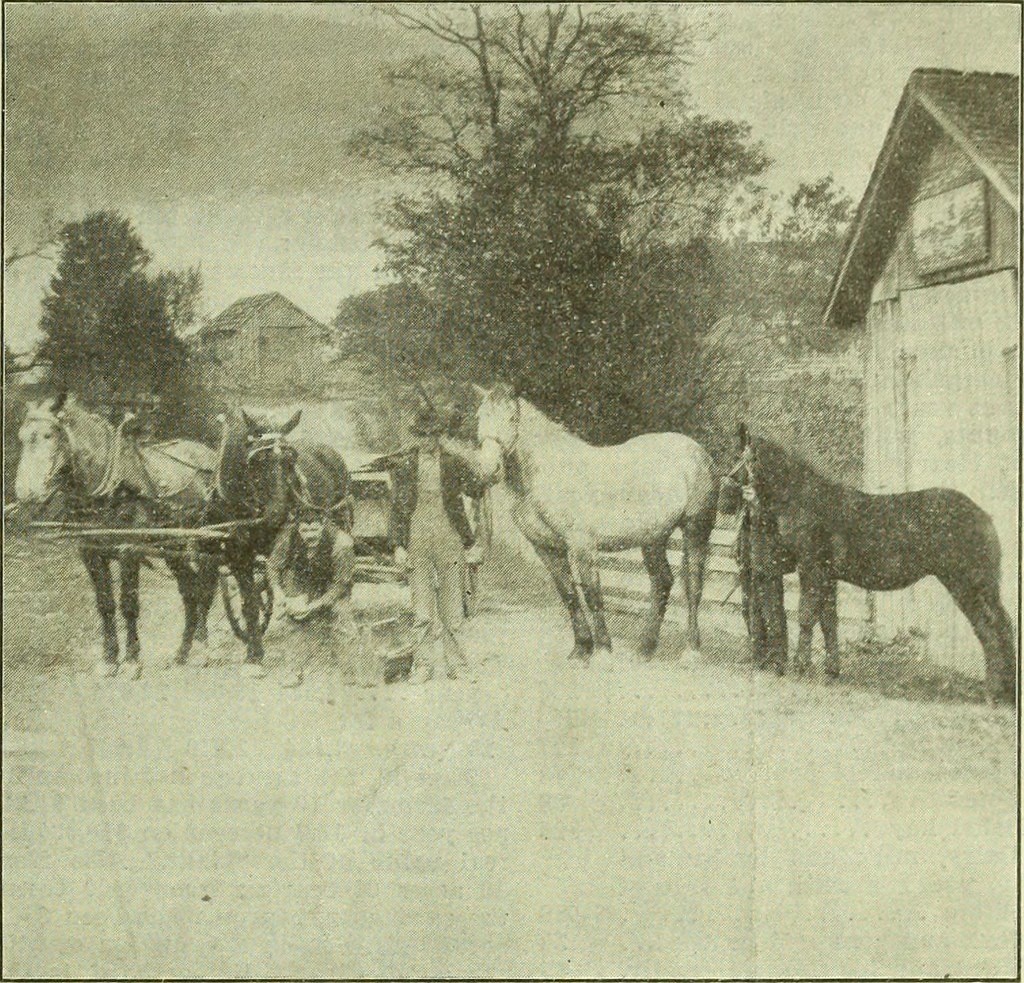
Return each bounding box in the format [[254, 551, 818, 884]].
[[10, 350, 495, 688]]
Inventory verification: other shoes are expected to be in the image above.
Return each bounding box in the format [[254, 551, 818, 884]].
[[409, 667, 436, 684], [447, 668, 475, 684]]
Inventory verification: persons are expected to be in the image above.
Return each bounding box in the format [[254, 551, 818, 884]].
[[262, 507, 361, 689], [385, 411, 506, 684], [717, 442, 796, 674]]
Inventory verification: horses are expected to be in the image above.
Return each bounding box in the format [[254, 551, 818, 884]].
[[469, 382, 720, 663], [717, 422, 1016, 710], [14, 387, 219, 681], [205, 403, 355, 679]]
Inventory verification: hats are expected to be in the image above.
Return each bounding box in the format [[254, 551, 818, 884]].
[[410, 411, 443, 433]]
